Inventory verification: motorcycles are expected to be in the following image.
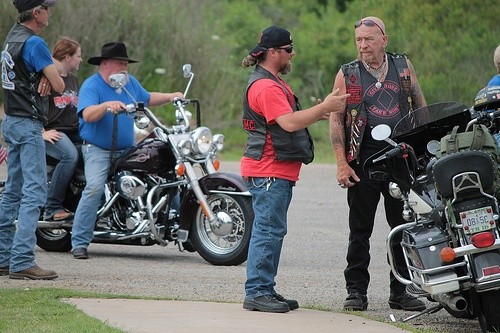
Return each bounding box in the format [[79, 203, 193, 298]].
[[361, 82, 500, 333], [12, 62, 255, 267]]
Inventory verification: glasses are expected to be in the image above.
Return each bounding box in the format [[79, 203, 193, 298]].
[[354, 19, 385, 36], [277, 43, 294, 54]]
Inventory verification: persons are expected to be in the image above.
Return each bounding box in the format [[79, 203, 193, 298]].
[[71, 43, 185, 258], [0, 0, 66, 280], [330, 16, 428, 311], [488, 44, 500, 87], [42, 39, 83, 221], [241, 25, 351, 312]]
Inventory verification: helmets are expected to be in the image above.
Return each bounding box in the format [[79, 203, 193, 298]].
[[472, 84, 499, 111]]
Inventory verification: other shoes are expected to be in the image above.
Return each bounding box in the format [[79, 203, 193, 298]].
[[273, 293, 300, 310], [8, 264, 59, 281], [53, 210, 73, 221], [73, 248, 88, 259], [0, 266, 10, 276], [343, 292, 369, 310], [243, 295, 290, 312], [387, 291, 427, 311]]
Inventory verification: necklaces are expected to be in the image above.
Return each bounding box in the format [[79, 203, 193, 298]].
[[363, 54, 386, 88]]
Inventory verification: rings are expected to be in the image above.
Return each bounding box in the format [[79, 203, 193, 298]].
[[338, 182, 341, 185], [341, 183, 344, 187], [42, 83, 47, 86], [46, 85, 48, 88]]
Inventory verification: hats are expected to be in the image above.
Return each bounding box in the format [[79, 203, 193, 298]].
[[86, 42, 141, 65], [12, 0, 57, 13], [247, 25, 293, 58]]
